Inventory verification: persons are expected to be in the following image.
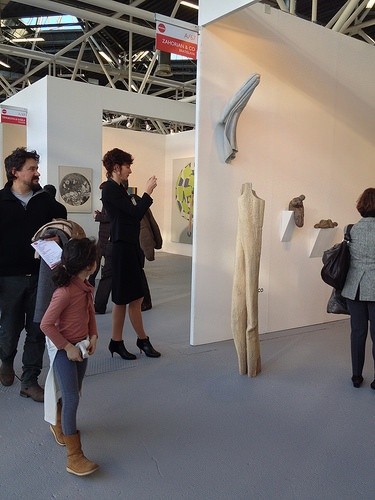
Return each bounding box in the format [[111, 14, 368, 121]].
[[326, 188, 375, 390], [40, 238, 101, 477], [0, 147, 69, 403], [92, 149, 163, 361], [92, 253, 155, 315], [84, 182, 117, 287]]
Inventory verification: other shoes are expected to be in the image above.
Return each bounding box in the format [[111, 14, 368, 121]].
[[371, 379, 375, 390], [94, 304, 105, 314], [351, 376, 363, 388], [141, 302, 152, 311]]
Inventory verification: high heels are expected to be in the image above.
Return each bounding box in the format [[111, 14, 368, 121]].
[[108, 338, 137, 360], [137, 336, 161, 357]]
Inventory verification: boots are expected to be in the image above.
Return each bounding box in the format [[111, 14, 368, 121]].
[[63, 430, 98, 476], [50, 403, 66, 446]]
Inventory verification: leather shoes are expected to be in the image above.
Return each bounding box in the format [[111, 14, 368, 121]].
[[20, 382, 44, 402], [0, 361, 15, 387]]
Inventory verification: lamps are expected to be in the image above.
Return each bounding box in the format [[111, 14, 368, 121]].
[[98, 51, 113, 63], [0, 55, 11, 69], [144, 121, 151, 131], [126, 119, 132, 128], [11, 38, 45, 42]]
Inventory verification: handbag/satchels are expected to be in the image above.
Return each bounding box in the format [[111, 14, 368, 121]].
[[327, 288, 350, 314], [321, 224, 356, 291]]
